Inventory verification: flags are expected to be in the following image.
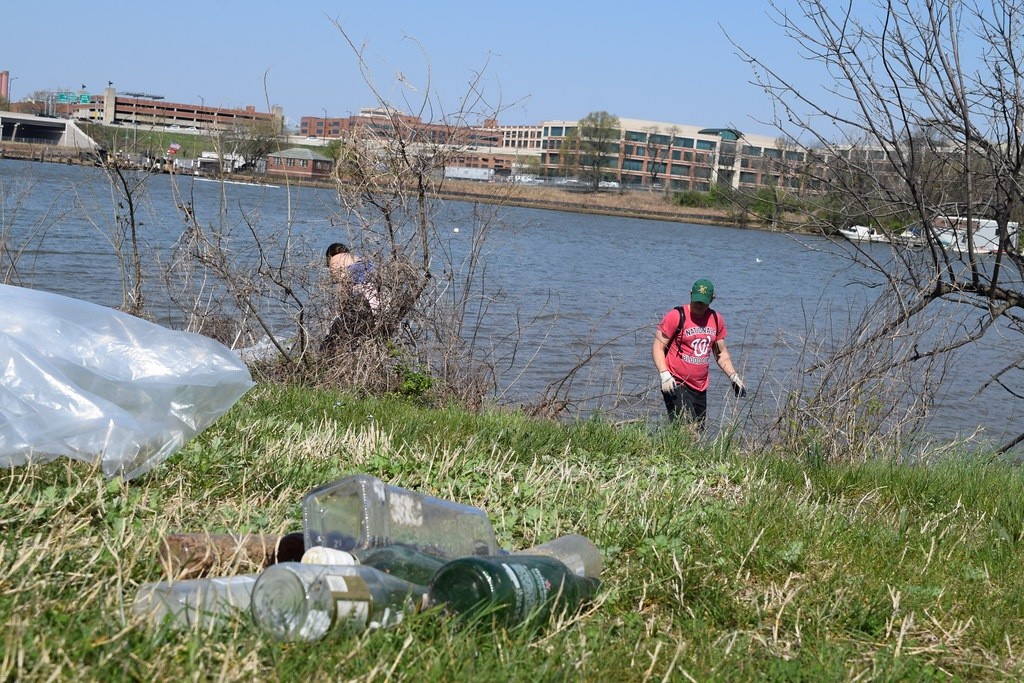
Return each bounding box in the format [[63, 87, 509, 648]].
[[170, 140, 181, 155]]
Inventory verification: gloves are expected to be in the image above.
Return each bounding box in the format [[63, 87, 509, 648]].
[[660, 370, 678, 399], [728, 371, 747, 397]]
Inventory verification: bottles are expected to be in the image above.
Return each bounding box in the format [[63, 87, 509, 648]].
[[133, 474, 604, 647]]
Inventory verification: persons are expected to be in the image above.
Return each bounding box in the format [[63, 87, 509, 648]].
[[651, 279, 747, 432], [319, 242, 383, 352]]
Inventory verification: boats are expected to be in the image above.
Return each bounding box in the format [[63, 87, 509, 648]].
[[839, 216, 1020, 258]]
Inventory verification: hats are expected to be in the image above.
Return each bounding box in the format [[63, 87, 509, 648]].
[[691, 279, 714, 305]]
